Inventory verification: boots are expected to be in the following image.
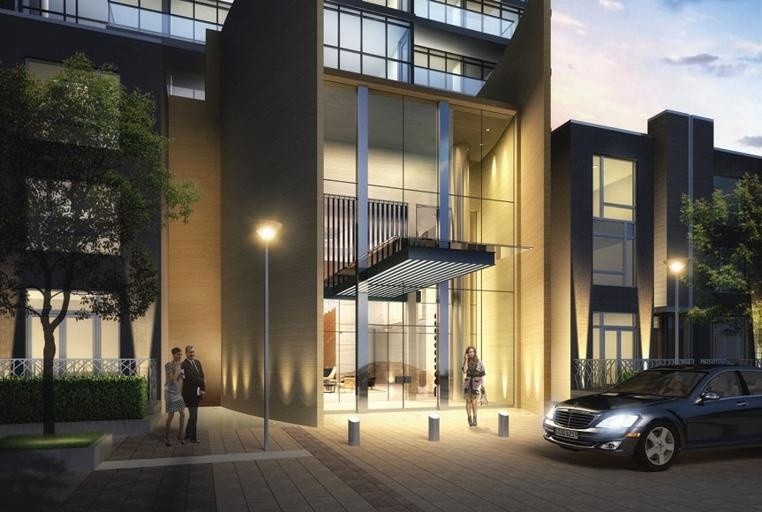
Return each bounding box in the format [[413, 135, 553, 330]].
[[468, 416, 477, 426]]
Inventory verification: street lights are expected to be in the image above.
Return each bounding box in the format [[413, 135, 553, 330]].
[[249, 217, 285, 455], [662, 255, 693, 365]]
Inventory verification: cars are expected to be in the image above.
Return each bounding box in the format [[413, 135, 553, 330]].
[[540, 362, 762, 468]]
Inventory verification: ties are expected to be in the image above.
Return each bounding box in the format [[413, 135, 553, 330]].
[[192, 361, 198, 375]]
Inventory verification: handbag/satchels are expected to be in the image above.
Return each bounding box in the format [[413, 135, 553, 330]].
[[479, 386, 489, 407]]
